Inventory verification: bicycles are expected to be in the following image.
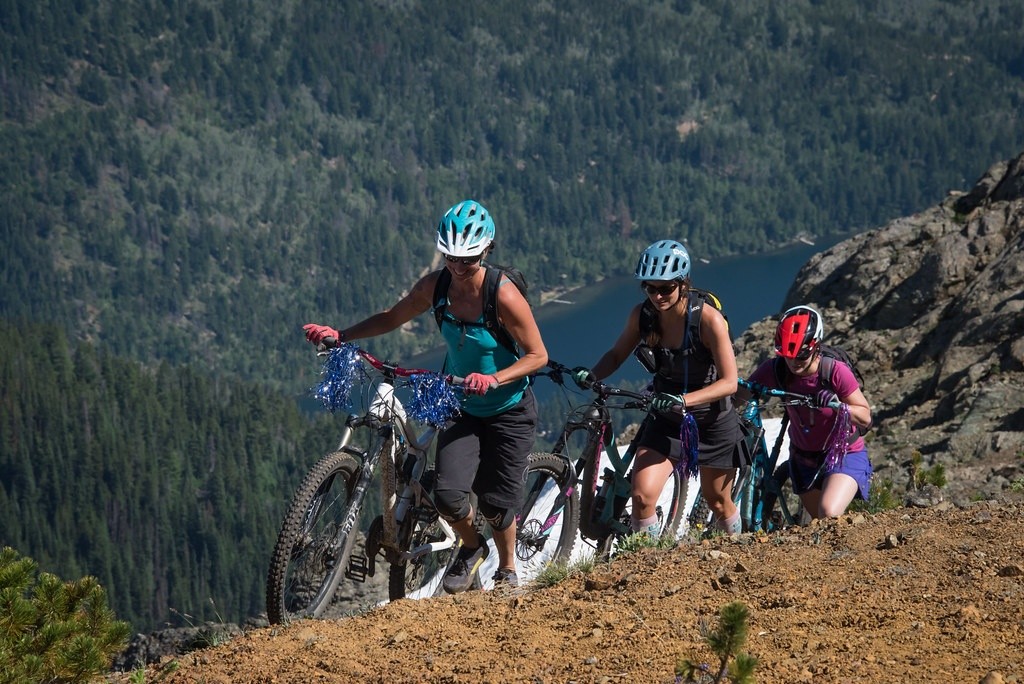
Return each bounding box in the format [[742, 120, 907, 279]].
[[689, 376, 847, 536], [470, 360, 688, 591], [264, 330, 470, 626]]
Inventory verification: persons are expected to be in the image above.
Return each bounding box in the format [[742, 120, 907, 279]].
[[571, 240, 744, 544], [302, 201, 549, 592], [733, 307, 874, 520]]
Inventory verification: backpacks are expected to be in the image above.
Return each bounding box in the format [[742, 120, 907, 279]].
[[772, 344, 873, 444], [639, 288, 741, 411], [432, 260, 533, 359]]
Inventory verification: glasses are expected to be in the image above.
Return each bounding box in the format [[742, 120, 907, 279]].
[[642, 283, 678, 295], [795, 349, 812, 361], [443, 254, 481, 264]]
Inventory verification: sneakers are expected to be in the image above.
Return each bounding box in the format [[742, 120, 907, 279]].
[[443, 533, 489, 595], [492, 568, 518, 588]]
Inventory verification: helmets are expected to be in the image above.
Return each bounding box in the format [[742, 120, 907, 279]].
[[633, 240, 691, 281], [773, 305, 824, 359], [434, 200, 496, 257]]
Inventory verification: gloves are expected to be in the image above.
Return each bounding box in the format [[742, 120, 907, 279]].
[[814, 388, 840, 408], [303, 324, 340, 346], [464, 372, 499, 396], [651, 392, 686, 414], [571, 366, 597, 389]]
